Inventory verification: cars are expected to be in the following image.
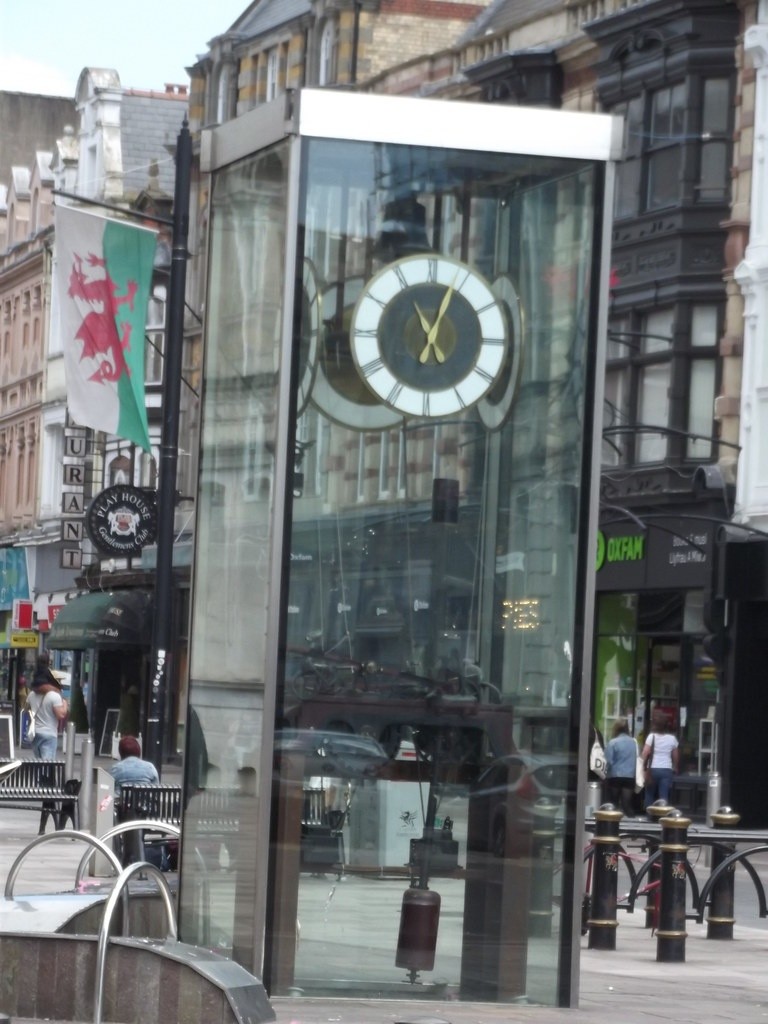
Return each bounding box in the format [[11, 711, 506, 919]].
[[228, 728, 392, 816], [442, 753, 573, 844]]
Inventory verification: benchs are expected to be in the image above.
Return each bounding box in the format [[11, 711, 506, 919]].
[[118, 783, 351, 882], [0, 756, 81, 840]]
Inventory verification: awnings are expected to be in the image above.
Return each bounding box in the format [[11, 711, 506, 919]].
[[48, 589, 152, 652]]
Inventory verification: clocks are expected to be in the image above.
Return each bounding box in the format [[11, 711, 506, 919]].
[[347, 255, 507, 417]]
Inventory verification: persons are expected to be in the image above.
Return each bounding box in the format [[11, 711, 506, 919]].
[[641, 719, 679, 808], [109, 737, 159, 811], [118, 685, 140, 736], [69, 672, 89, 733], [604, 720, 639, 841], [565, 715, 604, 782], [26, 654, 68, 833]]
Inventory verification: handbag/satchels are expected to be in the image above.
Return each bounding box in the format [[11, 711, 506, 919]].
[[589, 725, 607, 780], [645, 735, 655, 770], [634, 738, 645, 793], [22, 710, 37, 745]]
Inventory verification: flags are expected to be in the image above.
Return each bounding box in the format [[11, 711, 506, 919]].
[[53, 205, 156, 453]]
